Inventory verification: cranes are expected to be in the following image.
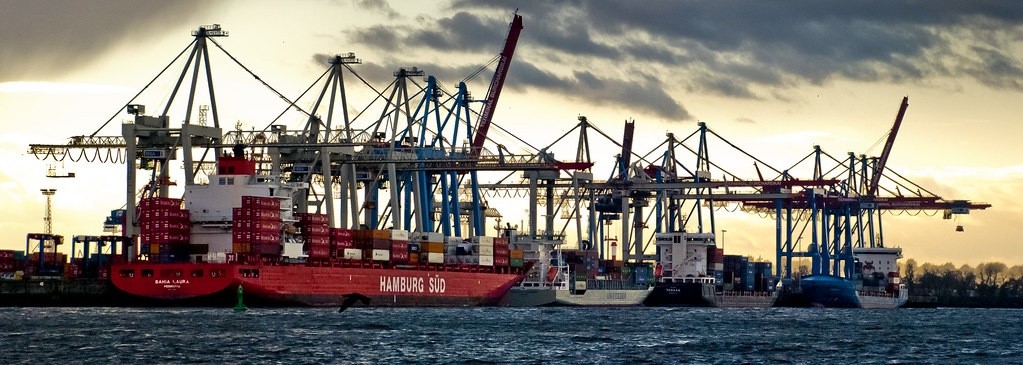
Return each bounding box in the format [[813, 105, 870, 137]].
[[26, 10, 992, 295]]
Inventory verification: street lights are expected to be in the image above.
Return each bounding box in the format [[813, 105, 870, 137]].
[[798, 236, 805, 293], [721, 230, 727, 253]]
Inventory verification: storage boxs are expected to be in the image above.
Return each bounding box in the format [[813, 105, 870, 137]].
[[0, 249, 82, 279], [855, 271, 900, 293], [545, 246, 653, 292], [714, 249, 776, 295], [98, 196, 526, 280]]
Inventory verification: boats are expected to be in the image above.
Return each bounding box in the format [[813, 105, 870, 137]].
[[91, 117, 656, 307]]
